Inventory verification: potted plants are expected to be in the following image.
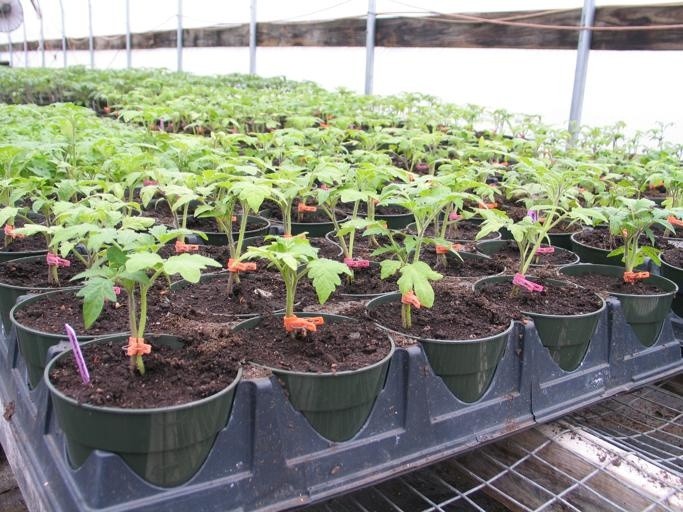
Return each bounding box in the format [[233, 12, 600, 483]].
[[0, 63, 682, 494]]
